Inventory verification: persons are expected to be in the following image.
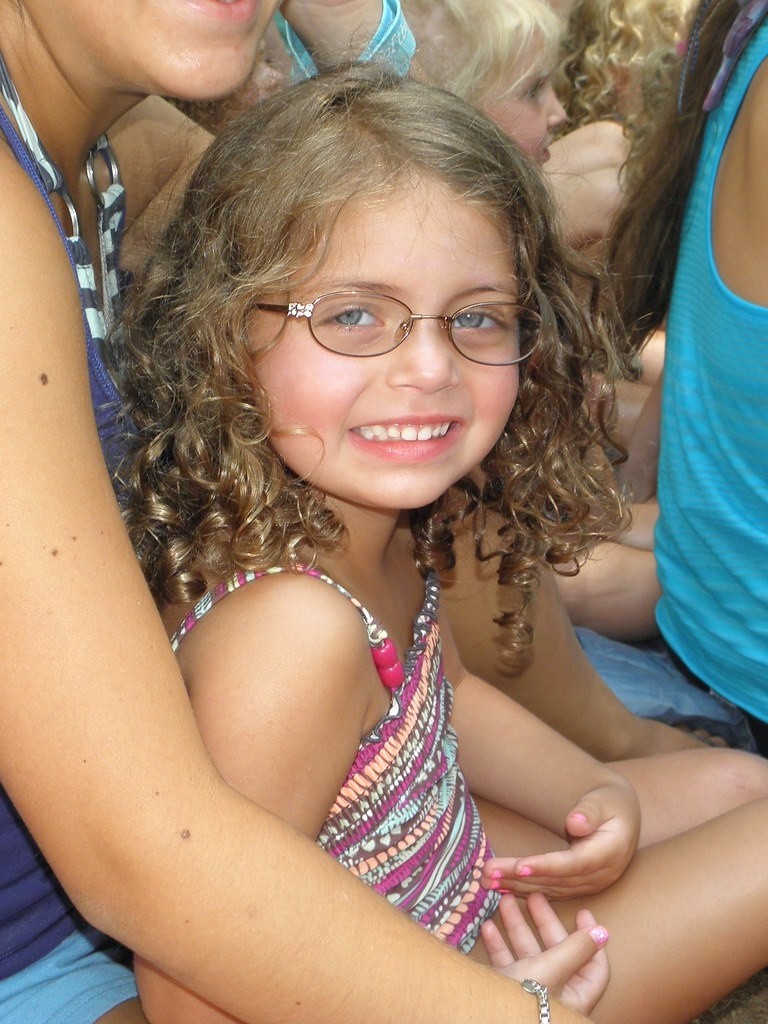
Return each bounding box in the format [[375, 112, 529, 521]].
[[0, 0, 768, 1024]]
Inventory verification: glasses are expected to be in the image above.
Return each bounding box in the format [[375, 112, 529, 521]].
[[245, 289, 570, 379]]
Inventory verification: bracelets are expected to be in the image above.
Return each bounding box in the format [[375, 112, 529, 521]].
[[520, 977, 551, 1024]]
